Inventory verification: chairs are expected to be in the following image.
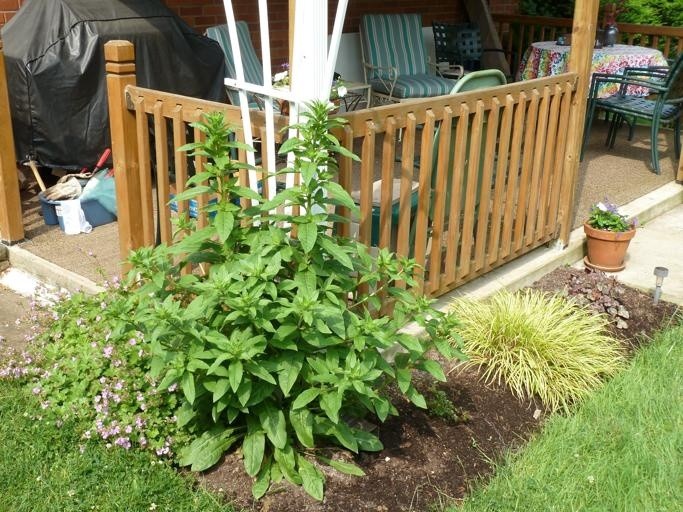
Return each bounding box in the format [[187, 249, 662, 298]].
[[352, 13, 459, 148], [331, 66, 510, 269], [206, 19, 291, 169], [579, 51, 683, 175], [428, 20, 513, 87]]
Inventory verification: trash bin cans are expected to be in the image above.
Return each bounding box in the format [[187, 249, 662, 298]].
[[347, 69, 507, 302]]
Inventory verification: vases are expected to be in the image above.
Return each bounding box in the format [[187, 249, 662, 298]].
[[274, 96, 339, 117], [584, 223, 635, 274]]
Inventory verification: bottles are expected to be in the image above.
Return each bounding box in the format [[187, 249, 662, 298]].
[[595, 24, 617, 48]]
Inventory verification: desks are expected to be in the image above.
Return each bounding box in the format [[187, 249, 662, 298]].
[[331, 79, 370, 113], [514, 41, 669, 144]]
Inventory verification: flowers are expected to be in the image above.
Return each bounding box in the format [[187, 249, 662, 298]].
[[271, 61, 352, 101], [586, 198, 639, 232]]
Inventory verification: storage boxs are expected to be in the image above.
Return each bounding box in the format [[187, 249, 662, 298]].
[[352, 185, 429, 263], [35, 187, 115, 234]]
